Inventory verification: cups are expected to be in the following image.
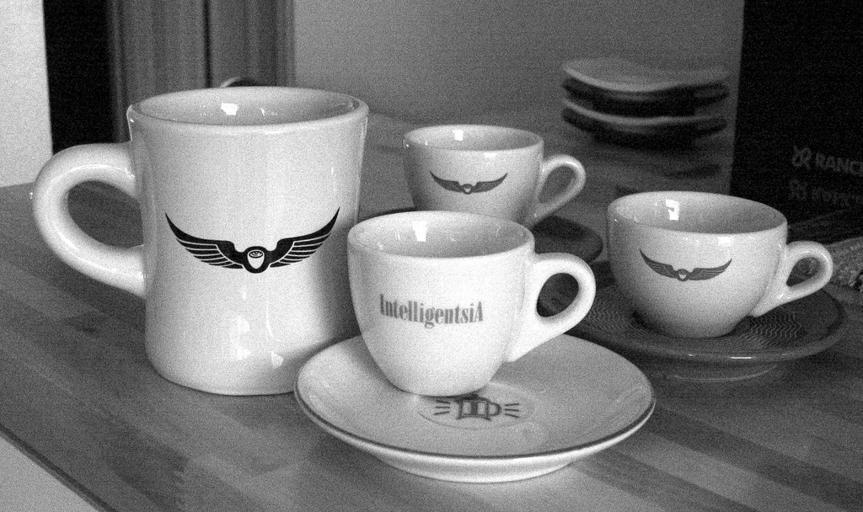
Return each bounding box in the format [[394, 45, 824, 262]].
[[402, 122, 586, 233], [607, 191, 835, 343], [344, 210, 597, 395], [30, 88, 370, 397]]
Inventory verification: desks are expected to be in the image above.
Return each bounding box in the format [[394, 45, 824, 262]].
[[0, 109, 861, 511]]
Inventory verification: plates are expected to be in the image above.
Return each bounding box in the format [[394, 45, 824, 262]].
[[541, 273, 847, 385], [293, 325, 659, 484], [562, 53, 730, 151], [361, 204, 602, 274]]
